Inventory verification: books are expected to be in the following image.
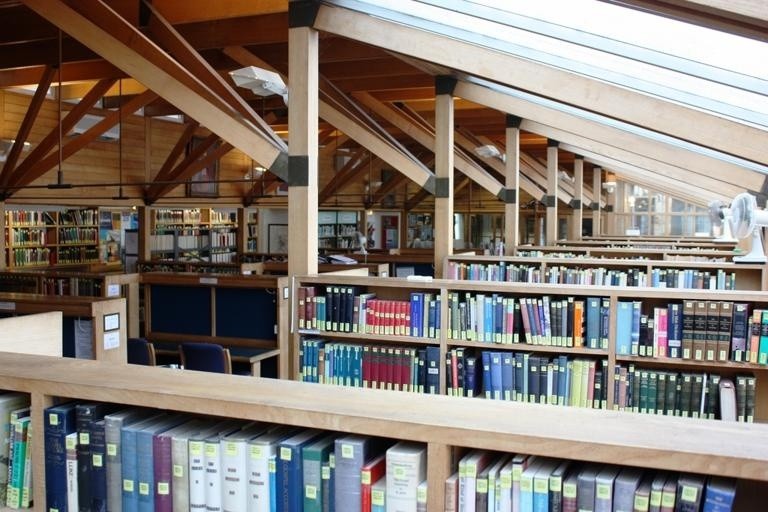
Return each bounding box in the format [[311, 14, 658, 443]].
[[1, 388, 737, 512], [299, 334, 440, 394], [318, 208, 441, 256], [614, 364, 757, 424], [2, 199, 140, 299], [446, 347, 608, 410], [448, 238, 739, 289], [298, 286, 441, 340], [615, 300, 768, 365], [447, 291, 610, 350], [147, 203, 288, 278]]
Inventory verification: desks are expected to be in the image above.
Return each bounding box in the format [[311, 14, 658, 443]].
[[139, 276, 287, 378]]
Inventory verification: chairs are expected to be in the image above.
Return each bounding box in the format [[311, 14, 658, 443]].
[[177, 342, 231, 375], [125, 337, 157, 367]]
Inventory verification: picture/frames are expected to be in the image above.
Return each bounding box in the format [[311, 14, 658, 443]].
[[267, 223, 288, 254], [186, 134, 220, 199]]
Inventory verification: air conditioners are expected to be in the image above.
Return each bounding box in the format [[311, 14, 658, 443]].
[[62, 109, 120, 142]]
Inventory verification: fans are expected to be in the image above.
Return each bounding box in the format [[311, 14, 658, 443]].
[[708, 197, 732, 243], [724, 191, 768, 268]]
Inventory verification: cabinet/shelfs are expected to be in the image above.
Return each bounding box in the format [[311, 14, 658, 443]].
[[124, 207, 238, 269], [4, 206, 99, 267], [441, 239, 766, 292], [0, 350, 766, 512], [288, 275, 766, 422], [0, 267, 141, 342], [136, 259, 264, 276], [318, 222, 357, 249]]
